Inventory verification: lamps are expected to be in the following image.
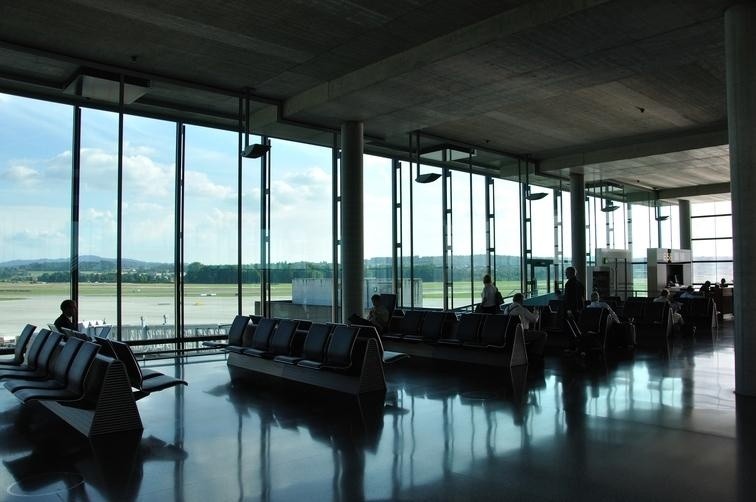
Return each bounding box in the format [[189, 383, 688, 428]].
[[600, 183, 621, 213], [523, 153, 548, 200], [414, 130, 442, 184], [655, 206, 670, 221], [237, 85, 271, 158]]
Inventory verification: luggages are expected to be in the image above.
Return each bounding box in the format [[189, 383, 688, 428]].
[[562, 314, 592, 363], [524, 305, 547, 364], [610, 322, 635, 358]]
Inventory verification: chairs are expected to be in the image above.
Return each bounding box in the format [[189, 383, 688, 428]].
[[199, 311, 408, 404], [391, 286, 734, 385], [0, 319, 178, 455]]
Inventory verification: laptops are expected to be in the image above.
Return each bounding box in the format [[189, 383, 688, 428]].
[[90, 327, 111, 342]]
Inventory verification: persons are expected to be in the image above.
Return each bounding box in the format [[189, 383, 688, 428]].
[[504, 293, 548, 365], [54, 300, 76, 337], [700, 281, 710, 291], [720, 278, 726, 288], [558, 267, 584, 337], [353, 295, 389, 336], [680, 285, 694, 298], [480, 275, 497, 313], [653, 289, 671, 305], [586, 292, 620, 325]]
